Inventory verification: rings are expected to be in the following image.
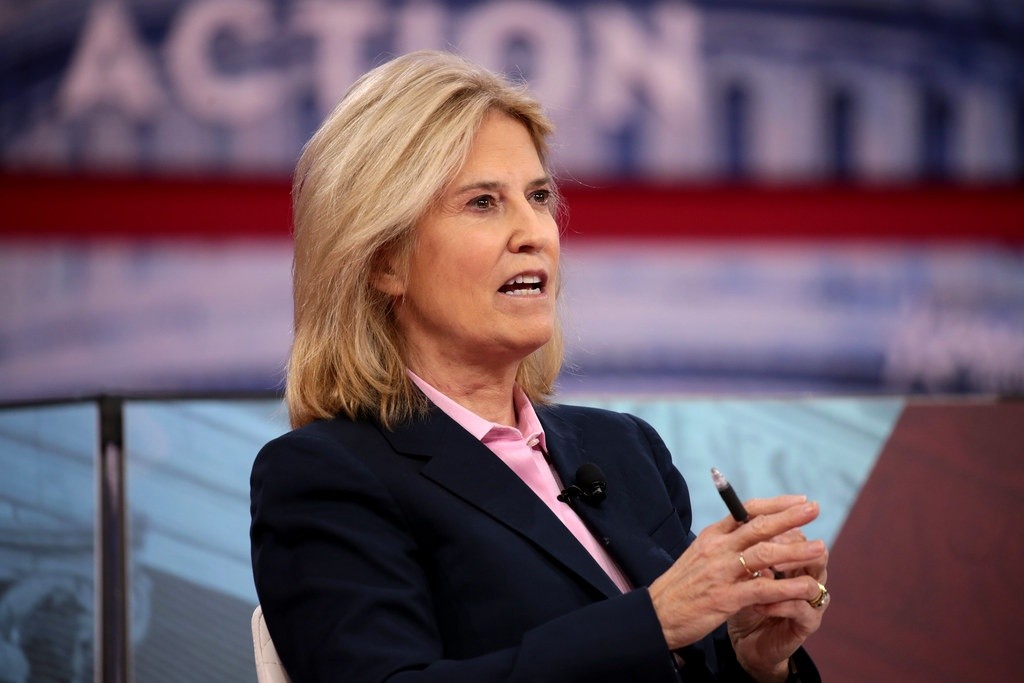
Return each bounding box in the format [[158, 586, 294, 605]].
[[739, 553, 762, 577], [810, 582, 828, 608]]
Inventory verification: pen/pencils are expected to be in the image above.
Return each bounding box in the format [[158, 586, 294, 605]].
[[710, 466, 785, 580]]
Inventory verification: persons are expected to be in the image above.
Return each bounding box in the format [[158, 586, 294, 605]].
[[250, 49, 830, 683]]
